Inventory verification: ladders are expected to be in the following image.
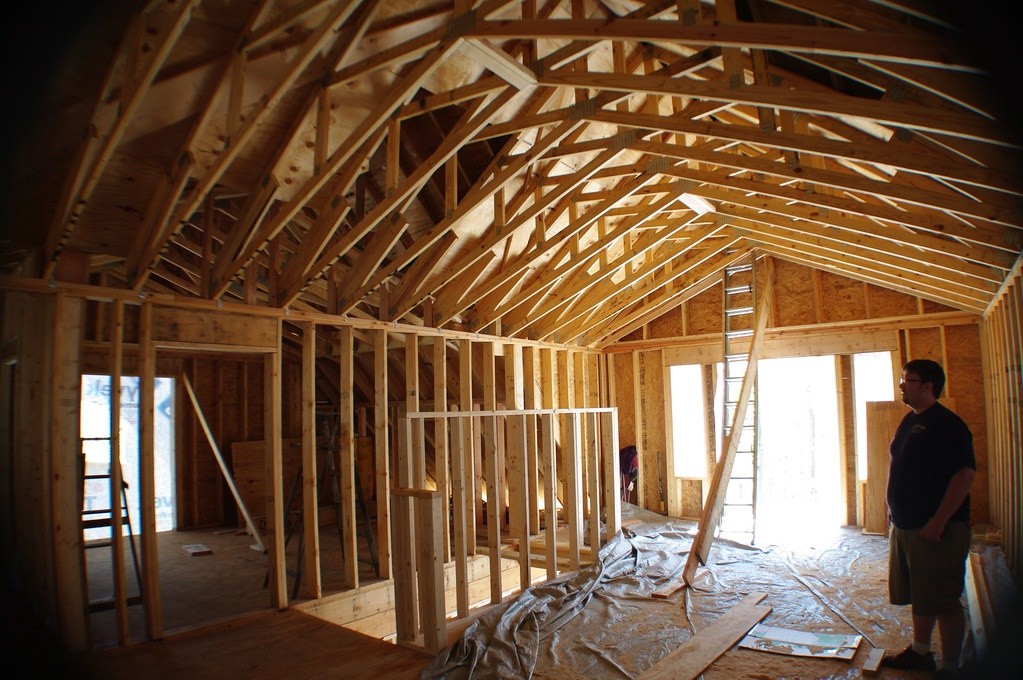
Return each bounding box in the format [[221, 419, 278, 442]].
[[717, 247, 759, 545], [80, 436, 147, 621]]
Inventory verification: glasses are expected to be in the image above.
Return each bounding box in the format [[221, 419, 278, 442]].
[[899, 377, 922, 385]]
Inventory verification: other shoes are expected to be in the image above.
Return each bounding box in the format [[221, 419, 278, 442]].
[[881, 644, 937, 671], [926, 667, 968, 680]]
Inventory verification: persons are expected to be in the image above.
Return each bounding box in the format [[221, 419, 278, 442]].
[[881, 359, 977, 680]]
